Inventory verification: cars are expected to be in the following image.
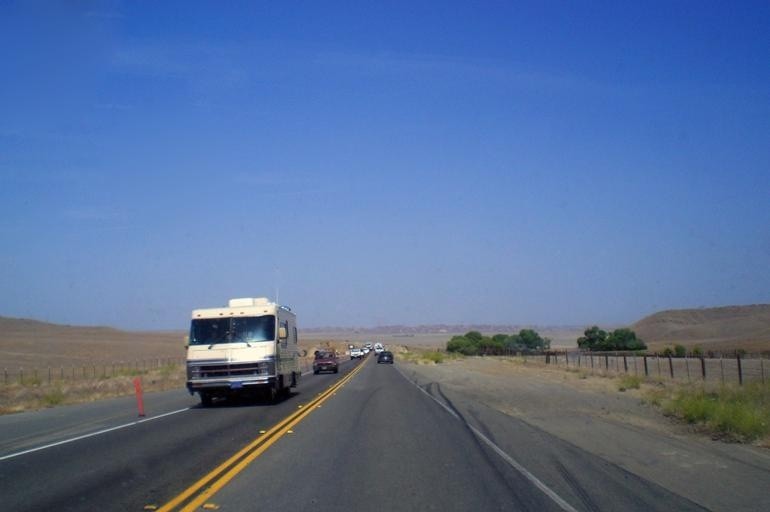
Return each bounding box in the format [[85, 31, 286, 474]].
[[374, 342, 385, 355], [350, 343, 372, 360], [378, 351, 394, 364]]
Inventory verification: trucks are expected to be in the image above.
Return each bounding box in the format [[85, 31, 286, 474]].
[[186, 296, 299, 404]]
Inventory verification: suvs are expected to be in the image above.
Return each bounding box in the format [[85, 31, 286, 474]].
[[314, 350, 338, 374]]
